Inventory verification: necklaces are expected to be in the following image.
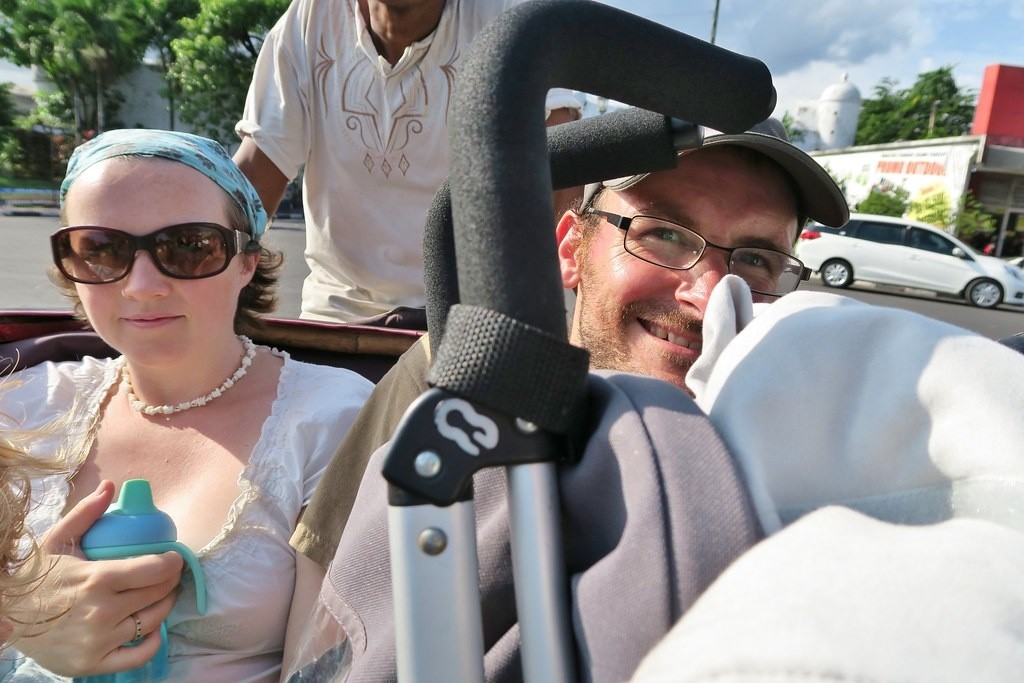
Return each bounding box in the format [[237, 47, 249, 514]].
[[120, 333, 258, 417]]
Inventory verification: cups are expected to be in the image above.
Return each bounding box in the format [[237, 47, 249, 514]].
[[70, 480, 205, 682]]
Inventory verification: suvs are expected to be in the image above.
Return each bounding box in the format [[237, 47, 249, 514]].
[[786, 214, 1024, 310]]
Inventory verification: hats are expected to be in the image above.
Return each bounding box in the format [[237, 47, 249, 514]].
[[588, 116, 851, 229]]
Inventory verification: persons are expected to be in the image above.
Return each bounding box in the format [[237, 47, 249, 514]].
[[279, 120, 851, 683], [230, 0, 537, 335], [0, 128, 376, 683]]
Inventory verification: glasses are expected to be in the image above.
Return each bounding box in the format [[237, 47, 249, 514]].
[[51, 221, 255, 284], [585, 207, 812, 297]]
